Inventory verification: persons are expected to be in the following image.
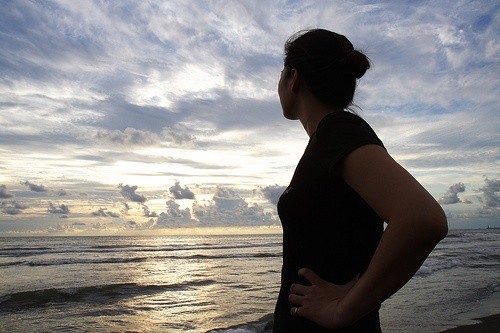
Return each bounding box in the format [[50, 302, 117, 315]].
[[271, 27, 449, 332]]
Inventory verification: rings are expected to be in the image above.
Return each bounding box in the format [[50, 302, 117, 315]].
[[294, 307, 298, 313]]
